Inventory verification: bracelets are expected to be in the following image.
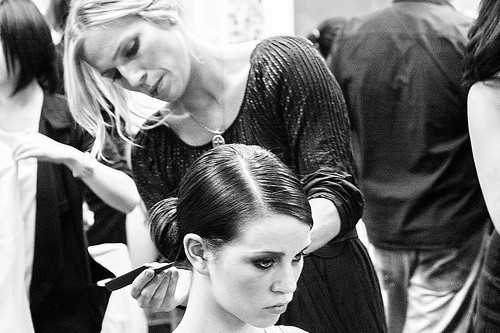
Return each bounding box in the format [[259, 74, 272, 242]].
[[73, 152, 94, 179]]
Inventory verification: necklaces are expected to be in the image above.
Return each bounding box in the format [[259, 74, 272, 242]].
[[188, 73, 228, 148]]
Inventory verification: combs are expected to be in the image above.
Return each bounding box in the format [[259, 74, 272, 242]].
[[104, 260, 177, 292]]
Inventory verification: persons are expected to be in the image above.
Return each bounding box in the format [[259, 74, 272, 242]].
[[0, 0, 141, 333], [63, 1, 389, 333], [143, 142, 314, 333], [326, 0, 489, 333], [459, 0, 500, 333]]
